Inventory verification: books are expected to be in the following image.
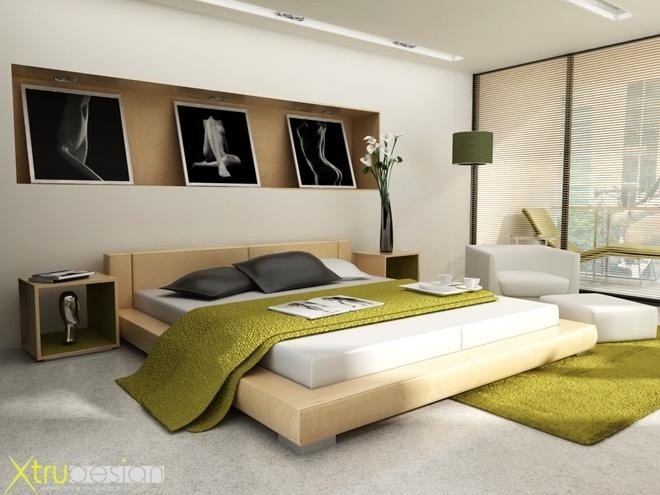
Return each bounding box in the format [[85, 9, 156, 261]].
[[29, 268, 93, 283], [268, 294, 383, 321]]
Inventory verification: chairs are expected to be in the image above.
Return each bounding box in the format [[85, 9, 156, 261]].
[[462, 241, 581, 303], [513, 206, 659, 285]]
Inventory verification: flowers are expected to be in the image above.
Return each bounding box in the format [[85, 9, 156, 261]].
[[359, 126, 408, 195]]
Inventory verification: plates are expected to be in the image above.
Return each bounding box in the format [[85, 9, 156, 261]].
[[398, 281, 484, 297]]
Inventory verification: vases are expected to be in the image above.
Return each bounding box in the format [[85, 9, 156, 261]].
[[377, 193, 395, 254]]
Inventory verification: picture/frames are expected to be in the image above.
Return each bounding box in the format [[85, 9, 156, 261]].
[[20, 80, 134, 185], [284, 111, 359, 188], [173, 97, 262, 187]]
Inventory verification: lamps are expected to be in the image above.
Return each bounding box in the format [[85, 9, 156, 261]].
[[451, 129, 494, 243]]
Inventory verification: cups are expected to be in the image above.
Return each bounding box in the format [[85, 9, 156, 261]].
[[440, 273, 452, 287], [463, 277, 480, 288]]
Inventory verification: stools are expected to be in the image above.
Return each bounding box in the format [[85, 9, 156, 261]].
[[540, 293, 660, 342]]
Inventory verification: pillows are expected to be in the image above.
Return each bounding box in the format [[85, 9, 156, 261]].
[[232, 251, 337, 294], [160, 264, 254, 300], [318, 256, 371, 280]]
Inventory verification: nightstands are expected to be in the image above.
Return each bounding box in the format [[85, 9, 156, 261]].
[[352, 250, 422, 281], [17, 269, 120, 364]]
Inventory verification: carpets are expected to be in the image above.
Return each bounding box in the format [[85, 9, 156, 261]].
[[450, 326, 660, 446]]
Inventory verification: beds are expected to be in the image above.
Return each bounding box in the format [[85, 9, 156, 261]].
[[102, 242, 599, 458]]
